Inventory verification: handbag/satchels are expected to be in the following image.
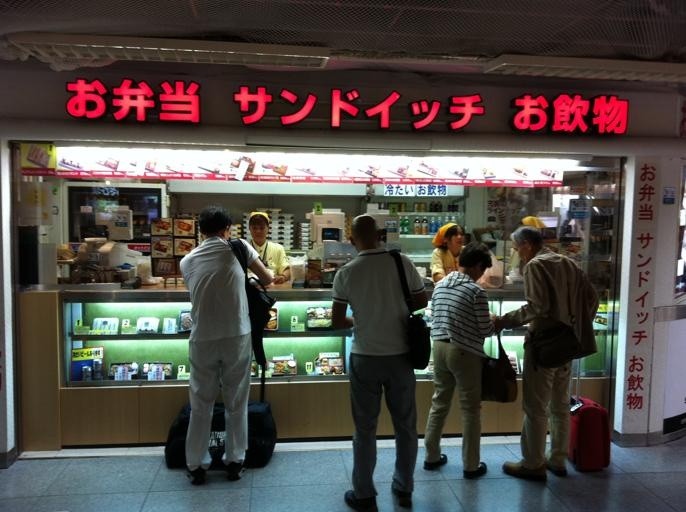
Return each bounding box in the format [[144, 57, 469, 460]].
[[165, 401, 277, 469], [403, 313, 430, 369], [481, 356, 517, 402], [531, 324, 582, 368]]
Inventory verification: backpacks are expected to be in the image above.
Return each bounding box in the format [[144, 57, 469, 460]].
[[226, 238, 277, 364]]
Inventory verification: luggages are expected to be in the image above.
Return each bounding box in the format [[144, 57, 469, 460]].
[[568, 359, 610, 473]]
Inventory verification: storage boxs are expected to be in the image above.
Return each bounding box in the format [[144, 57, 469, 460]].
[[150, 216, 196, 278]]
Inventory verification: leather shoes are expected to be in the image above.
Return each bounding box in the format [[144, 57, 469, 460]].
[[463, 462, 487, 479], [345, 490, 377, 512], [547, 465, 567, 476], [392, 488, 411, 508], [503, 462, 546, 481], [424, 454, 446, 470]]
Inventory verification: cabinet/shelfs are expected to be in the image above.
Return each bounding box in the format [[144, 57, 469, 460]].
[[397, 211, 461, 262], [61, 283, 611, 446]]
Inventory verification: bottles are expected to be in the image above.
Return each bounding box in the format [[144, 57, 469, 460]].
[[400, 216, 429, 235], [82, 366, 93, 382], [92, 359, 103, 381], [429, 216, 456, 233], [429, 200, 443, 212]]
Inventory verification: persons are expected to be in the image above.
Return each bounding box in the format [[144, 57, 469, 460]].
[[509, 216, 546, 276], [245, 211, 292, 292], [330, 215, 428, 512], [179, 205, 276, 485], [492, 225, 600, 481], [422, 240, 498, 479], [429, 223, 467, 285]]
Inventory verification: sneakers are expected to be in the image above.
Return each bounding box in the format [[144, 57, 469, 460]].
[[224, 462, 246, 481], [185, 467, 206, 485]]
